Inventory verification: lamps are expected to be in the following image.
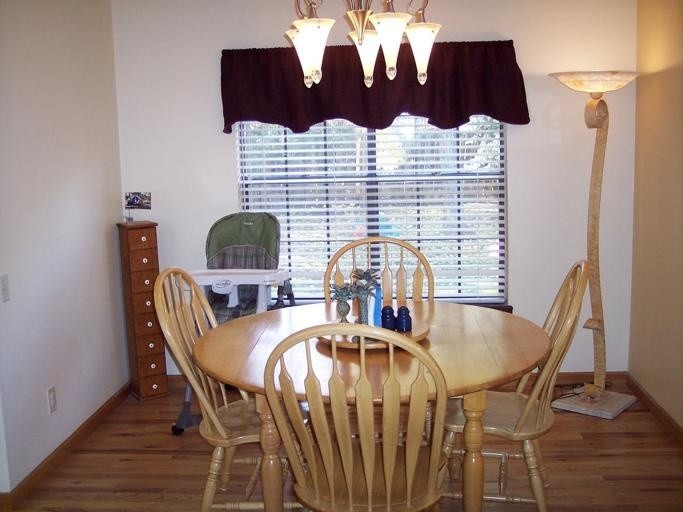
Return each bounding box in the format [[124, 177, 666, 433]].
[[285, 0, 442, 89], [547, 72, 640, 420]]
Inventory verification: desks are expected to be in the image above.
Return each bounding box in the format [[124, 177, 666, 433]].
[[192, 300, 552, 512]]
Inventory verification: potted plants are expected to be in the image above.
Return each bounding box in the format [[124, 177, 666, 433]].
[[329, 268, 381, 325]]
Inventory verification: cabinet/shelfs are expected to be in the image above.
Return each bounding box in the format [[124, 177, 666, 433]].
[[116, 220, 172, 401]]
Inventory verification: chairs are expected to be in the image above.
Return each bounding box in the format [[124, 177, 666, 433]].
[[323, 236, 434, 303], [263, 324, 447, 512], [431, 260, 588, 512], [153, 267, 310, 512], [172, 213, 296, 434]]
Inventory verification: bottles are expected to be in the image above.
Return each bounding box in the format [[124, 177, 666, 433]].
[[396, 305, 411, 332], [381, 305, 395, 331]]
